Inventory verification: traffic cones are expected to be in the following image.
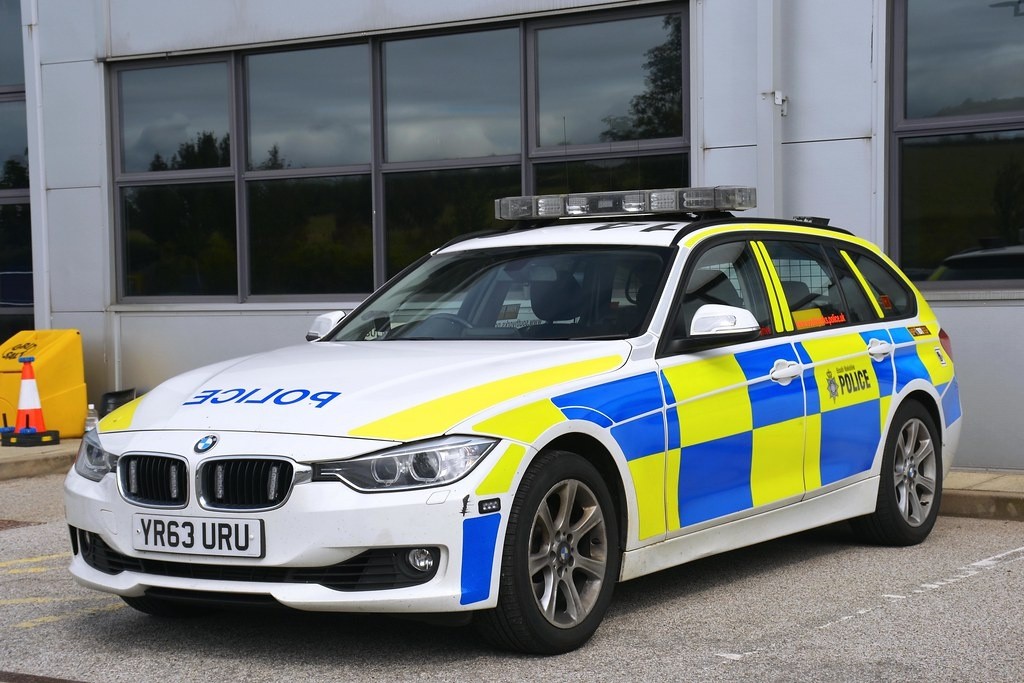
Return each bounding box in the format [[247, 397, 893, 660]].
[[12, 356, 48, 432]]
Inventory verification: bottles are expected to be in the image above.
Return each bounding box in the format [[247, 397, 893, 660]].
[[84, 405, 99, 435]]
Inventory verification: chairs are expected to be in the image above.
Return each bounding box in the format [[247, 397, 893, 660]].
[[517, 269, 592, 337], [636, 270, 741, 339]]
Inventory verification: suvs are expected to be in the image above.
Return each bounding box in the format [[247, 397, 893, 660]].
[[931, 243, 1024, 279], [62, 181, 964, 658]]
